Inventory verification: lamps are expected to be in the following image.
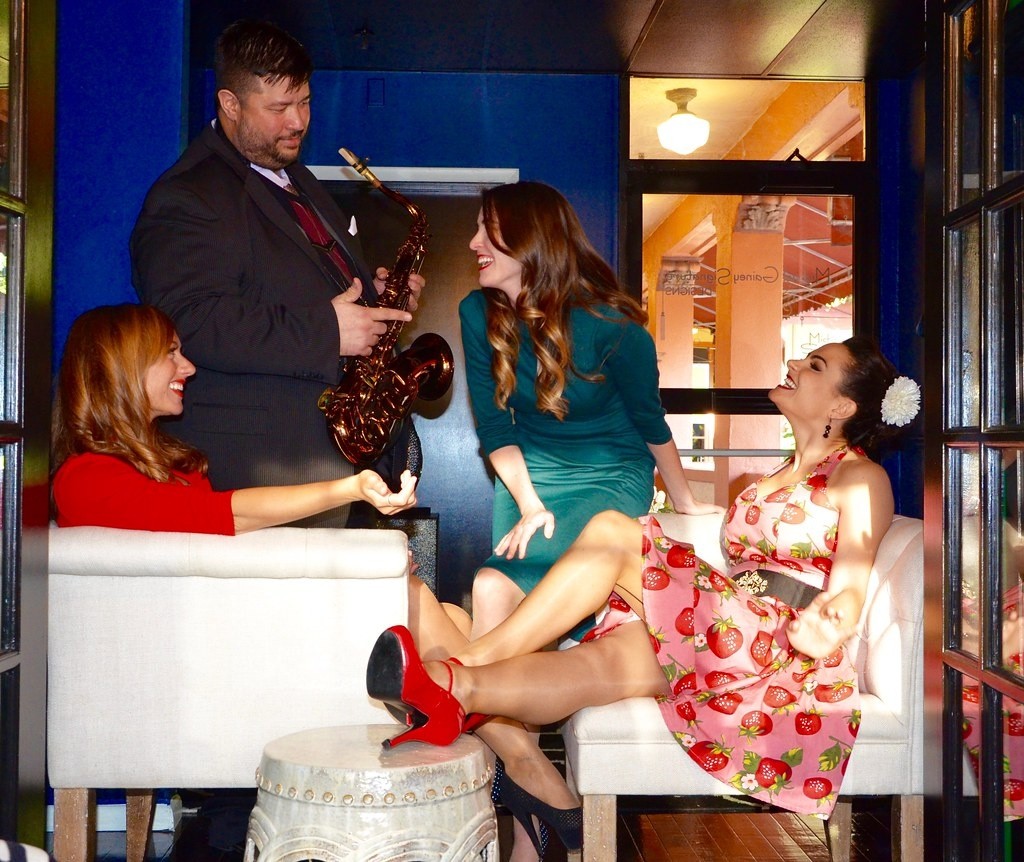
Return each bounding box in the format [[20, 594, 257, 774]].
[[657, 87, 709, 155]]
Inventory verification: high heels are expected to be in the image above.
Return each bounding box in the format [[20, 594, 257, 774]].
[[490, 761, 502, 803], [383, 656, 493, 734], [539, 818, 549, 862], [367, 626, 468, 751], [499, 772, 583, 858]]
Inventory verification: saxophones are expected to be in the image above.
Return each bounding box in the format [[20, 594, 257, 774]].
[[314, 146, 454, 466]]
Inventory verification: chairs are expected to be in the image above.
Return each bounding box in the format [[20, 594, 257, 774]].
[[47, 520, 407, 862], [556, 514, 923, 862]]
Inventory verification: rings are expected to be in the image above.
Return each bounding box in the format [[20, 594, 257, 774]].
[[388, 494, 393, 505]]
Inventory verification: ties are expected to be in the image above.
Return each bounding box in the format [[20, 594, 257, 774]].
[[282, 184, 297, 195]]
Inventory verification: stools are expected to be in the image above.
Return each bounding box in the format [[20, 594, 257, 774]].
[[243, 724, 500, 862]]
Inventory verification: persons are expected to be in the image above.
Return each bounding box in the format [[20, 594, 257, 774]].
[[48, 307, 583, 848], [130, 20, 424, 531], [369, 333, 922, 820], [960, 508, 1024, 822], [459, 182, 726, 862]]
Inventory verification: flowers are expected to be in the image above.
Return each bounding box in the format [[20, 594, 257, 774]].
[[880, 376, 921, 427]]
